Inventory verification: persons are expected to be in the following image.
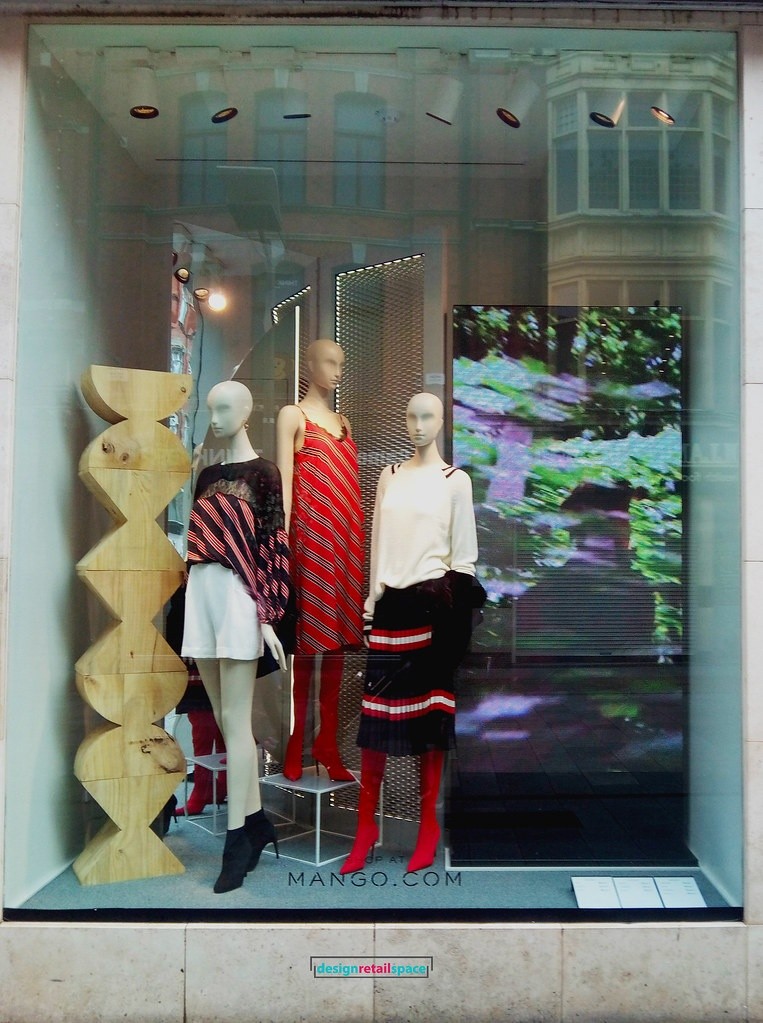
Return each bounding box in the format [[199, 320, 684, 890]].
[[180, 380, 291, 895], [276, 337, 364, 783], [336, 392, 481, 874]]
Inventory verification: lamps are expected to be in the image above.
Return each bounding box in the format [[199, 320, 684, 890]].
[[72, 39, 722, 128], [172, 253, 229, 312]]
